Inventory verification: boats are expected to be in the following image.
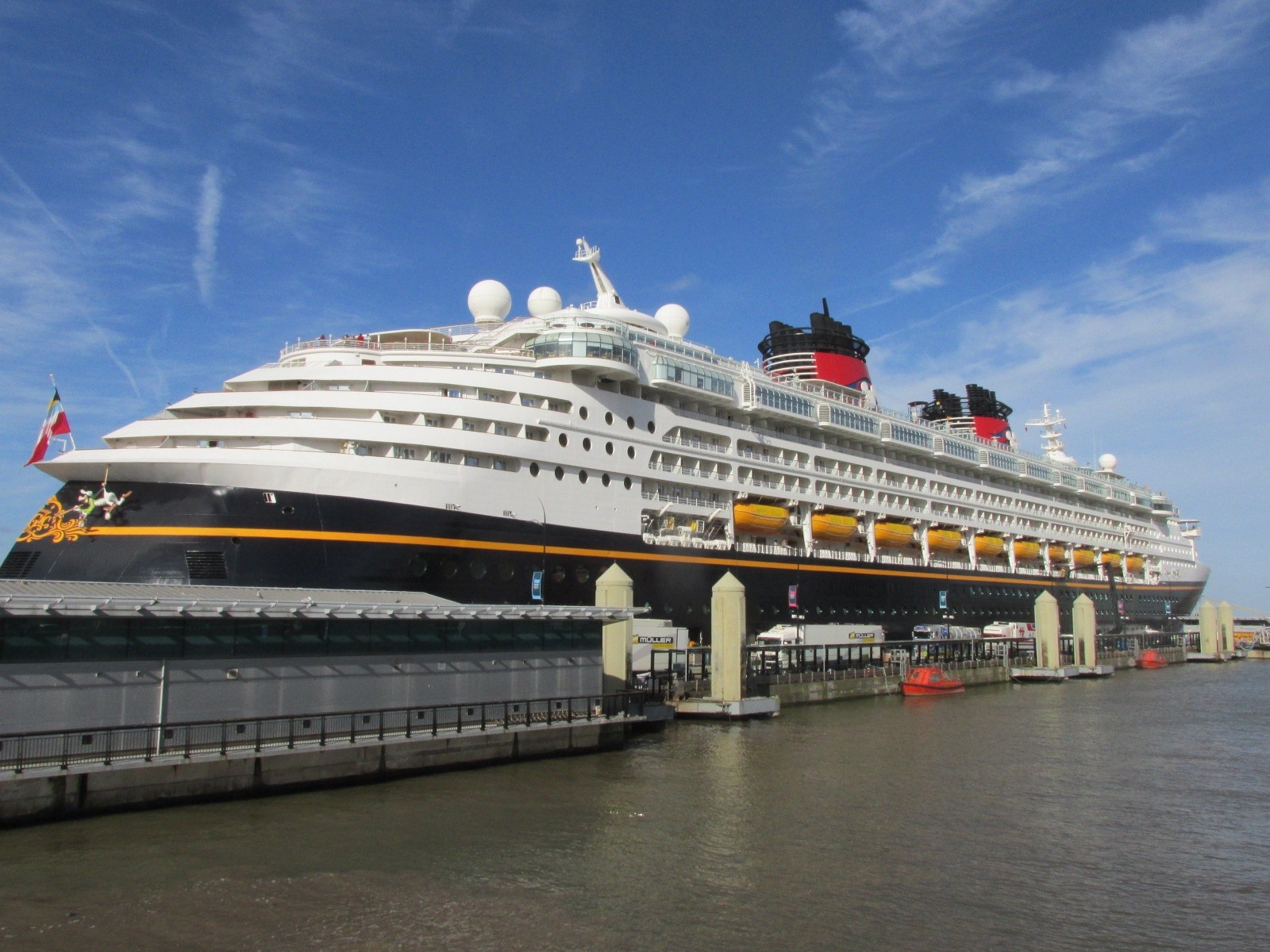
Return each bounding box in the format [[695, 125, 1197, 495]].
[[960, 536, 1003, 559], [999, 540, 1041, 559], [1100, 553, 1122, 568], [797, 510, 858, 542], [734, 501, 789, 533], [1048, 545, 1066, 562], [917, 528, 964, 554], [901, 668, 965, 695], [1072, 549, 1095, 567], [860, 520, 915, 547], [1135, 651, 1167, 670], [1126, 555, 1145, 571]]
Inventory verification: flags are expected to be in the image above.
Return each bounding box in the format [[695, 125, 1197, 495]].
[[789, 585, 797, 607], [1117, 600, 1125, 613], [23, 388, 71, 466], [1165, 601, 1171, 614], [531, 572, 542, 600], [939, 591, 946, 608]]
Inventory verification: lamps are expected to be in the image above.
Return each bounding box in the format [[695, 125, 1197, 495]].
[[756, 588, 1189, 617], [645, 602, 711, 616], [407, 554, 589, 583]]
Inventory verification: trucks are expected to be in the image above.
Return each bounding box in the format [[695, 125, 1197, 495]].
[[984, 622, 1035, 657], [755, 622, 884, 673], [912, 624, 983, 663]]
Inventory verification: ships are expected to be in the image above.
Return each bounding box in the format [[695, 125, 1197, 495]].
[[0, 239, 1207, 649]]
